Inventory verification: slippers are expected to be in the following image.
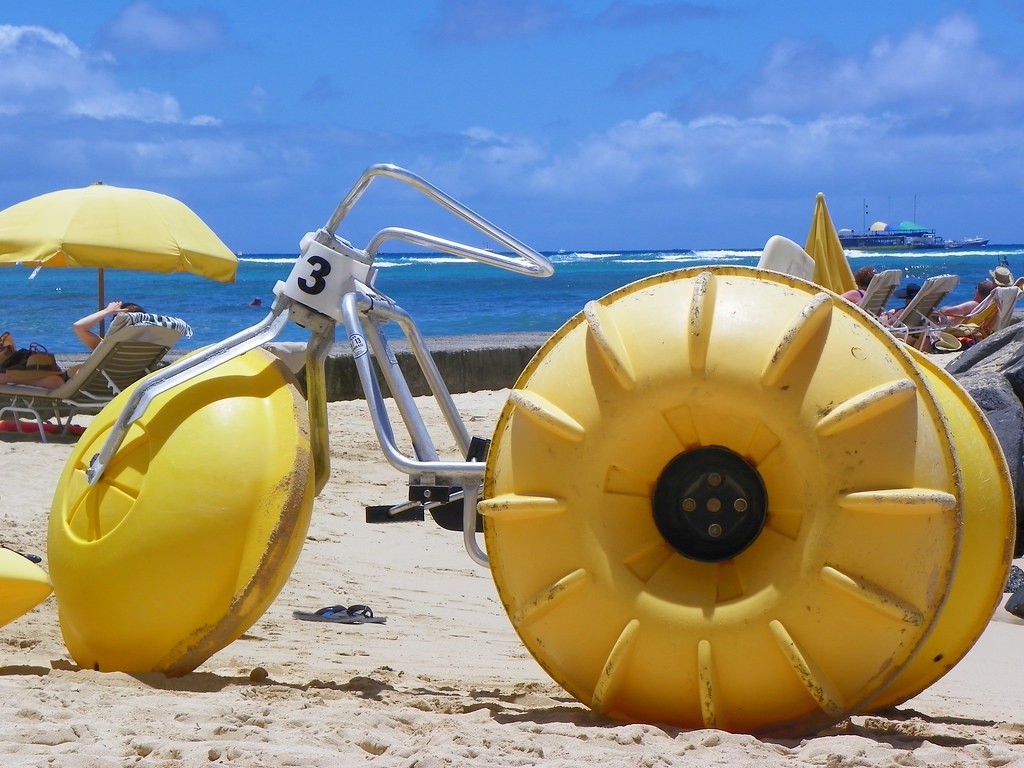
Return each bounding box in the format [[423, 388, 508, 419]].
[[292, 604, 365, 622], [348, 605, 386, 622]]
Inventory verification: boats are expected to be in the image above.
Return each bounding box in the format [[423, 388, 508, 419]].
[[945, 235, 989, 249], [838, 193, 945, 249]]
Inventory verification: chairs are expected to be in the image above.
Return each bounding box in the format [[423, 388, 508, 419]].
[[885, 271, 964, 351], [0, 312, 193, 443], [858, 269, 909, 345], [932, 277, 1024, 346]]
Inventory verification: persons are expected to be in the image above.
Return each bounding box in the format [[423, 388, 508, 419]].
[[0, 301, 146, 389], [840, 267, 877, 304], [250, 298, 262, 306], [932, 280, 997, 326], [881, 283, 921, 325], [988, 266, 1014, 286]]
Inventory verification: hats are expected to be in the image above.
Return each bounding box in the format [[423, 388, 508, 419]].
[[894, 283, 921, 299], [989, 266, 1015, 286], [932, 332, 962, 351], [6, 354, 64, 381]]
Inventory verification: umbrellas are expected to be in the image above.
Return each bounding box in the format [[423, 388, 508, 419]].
[[0, 182, 238, 340], [803, 192, 858, 296]]
